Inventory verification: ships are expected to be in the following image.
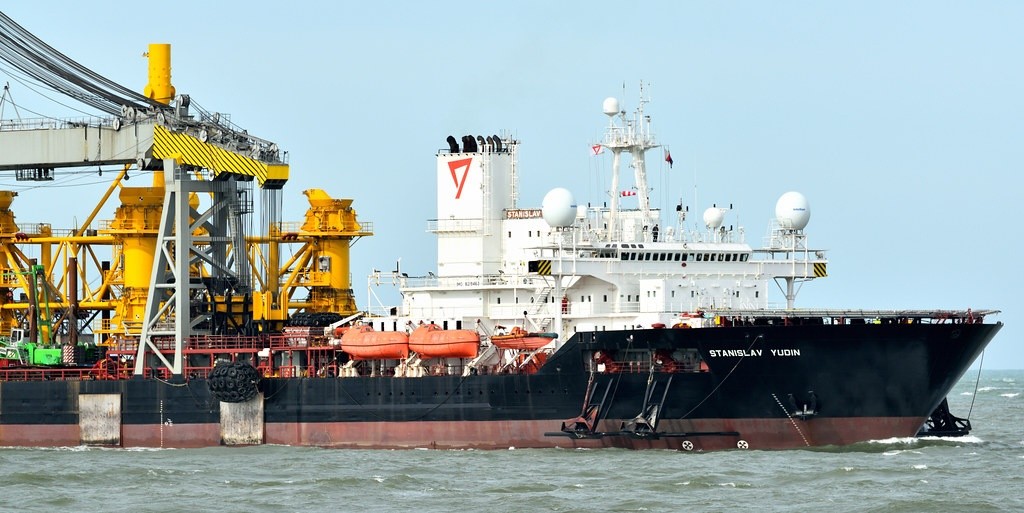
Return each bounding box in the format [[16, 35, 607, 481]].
[[0, 5, 1007, 456]]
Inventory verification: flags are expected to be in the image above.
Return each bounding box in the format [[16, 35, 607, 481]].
[[590, 144, 605, 155], [664, 146, 673, 168]]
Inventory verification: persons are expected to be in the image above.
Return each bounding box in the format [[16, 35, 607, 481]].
[[652, 223, 659, 242], [562, 295, 569, 314], [720, 225, 726, 241], [714, 312, 721, 326], [966, 307, 974, 324]]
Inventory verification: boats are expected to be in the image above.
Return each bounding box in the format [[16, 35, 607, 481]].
[[408, 324, 479, 360], [343, 325, 410, 363], [490, 332, 560, 350]]
